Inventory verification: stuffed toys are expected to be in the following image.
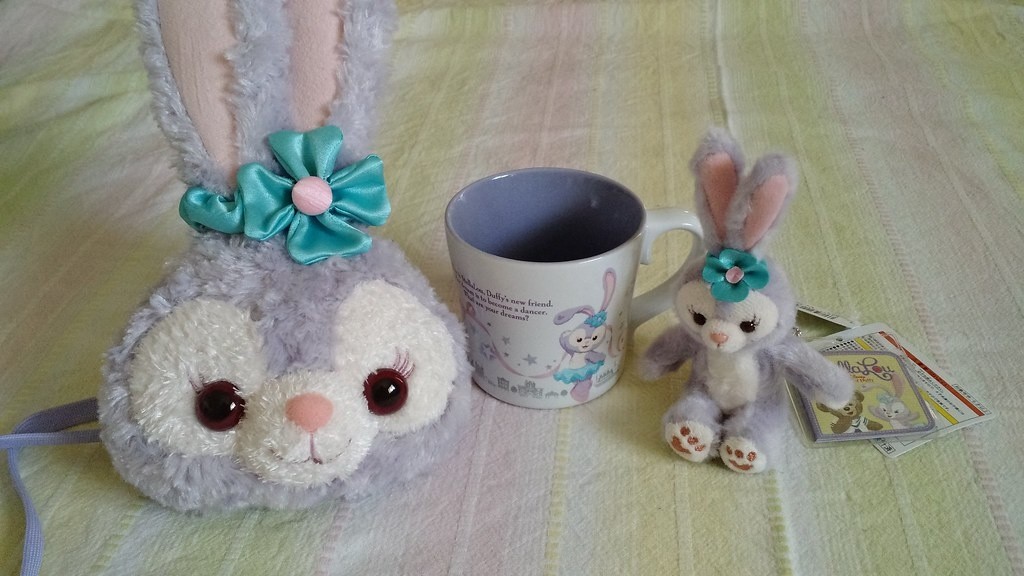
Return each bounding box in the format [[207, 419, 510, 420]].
[[633, 127, 855, 473], [97, 1, 476, 519]]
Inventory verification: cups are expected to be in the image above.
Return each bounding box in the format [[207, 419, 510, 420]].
[[444, 168, 705, 409]]
